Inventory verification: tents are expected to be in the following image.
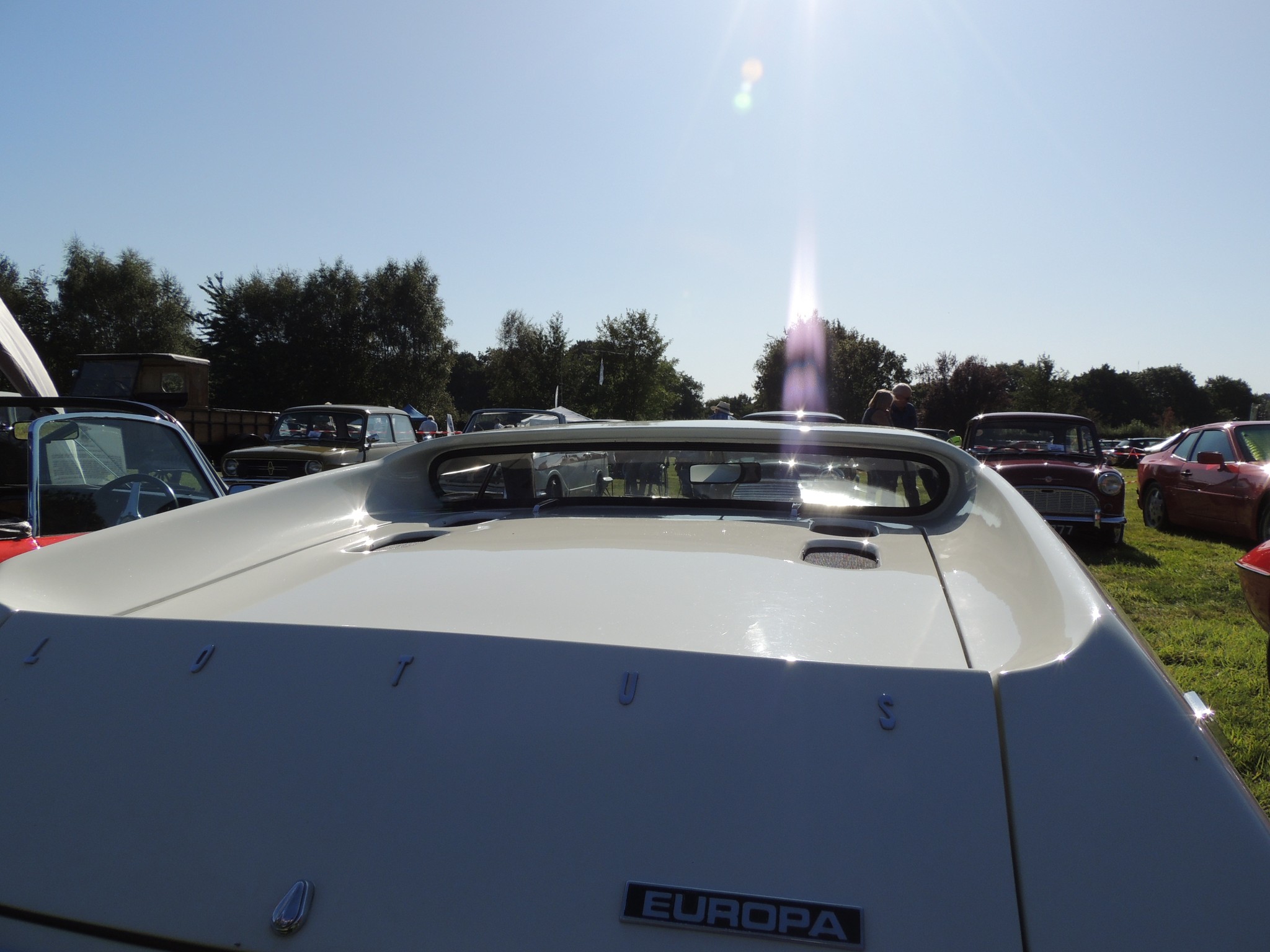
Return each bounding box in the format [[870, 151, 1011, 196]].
[[401, 403, 427, 421]]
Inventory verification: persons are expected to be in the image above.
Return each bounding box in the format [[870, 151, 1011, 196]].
[[625, 401, 737, 496], [947, 429, 962, 446], [316, 402, 334, 428], [861, 383, 921, 506], [492, 418, 504, 429], [419, 415, 438, 439]]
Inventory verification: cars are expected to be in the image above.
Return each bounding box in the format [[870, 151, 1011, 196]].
[[0, 394, 262, 563], [963, 412, 1130, 550], [217, 403, 423, 495], [914, 426, 950, 443], [1136, 420, 1270, 547], [1088, 437, 1168, 467], [710, 412, 862, 503], [435, 409, 610, 507]]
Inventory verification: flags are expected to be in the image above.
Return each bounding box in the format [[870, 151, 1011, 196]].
[[447, 414, 455, 436]]
